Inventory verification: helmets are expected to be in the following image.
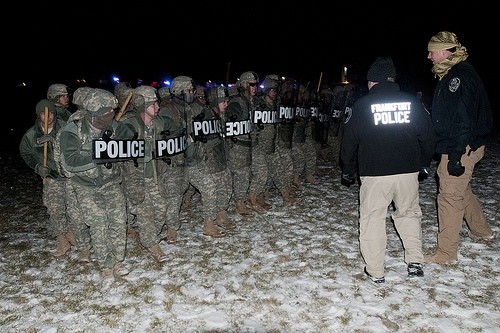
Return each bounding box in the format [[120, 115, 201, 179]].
[[72, 87, 93, 105], [47, 84, 68, 100], [36, 99, 56, 116], [114, 82, 131, 97], [119, 89, 135, 108], [130, 85, 158, 113], [83, 88, 118, 111], [158, 87, 170, 100], [170, 76, 193, 97], [195, 71, 356, 99]]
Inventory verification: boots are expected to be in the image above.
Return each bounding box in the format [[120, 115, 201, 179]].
[[128, 193, 270, 262], [283, 167, 323, 204], [54, 231, 128, 278]]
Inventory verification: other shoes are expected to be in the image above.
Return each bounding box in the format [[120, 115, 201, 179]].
[[407, 265, 424, 278], [468, 230, 496, 242], [364, 266, 385, 283], [429, 255, 459, 267]]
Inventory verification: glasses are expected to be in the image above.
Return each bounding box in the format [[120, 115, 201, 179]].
[[249, 83, 259, 87]]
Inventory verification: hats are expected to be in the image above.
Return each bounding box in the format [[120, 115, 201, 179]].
[[367, 58, 395, 82]]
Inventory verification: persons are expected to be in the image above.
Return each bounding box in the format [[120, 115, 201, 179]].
[[19, 72, 360, 277], [340, 56, 436, 284], [427, 31, 496, 265]]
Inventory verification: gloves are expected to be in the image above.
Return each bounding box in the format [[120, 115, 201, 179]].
[[418, 167, 428, 181], [341, 172, 356, 187], [39, 135, 54, 144], [34, 163, 51, 177], [447, 160, 465, 176]]
